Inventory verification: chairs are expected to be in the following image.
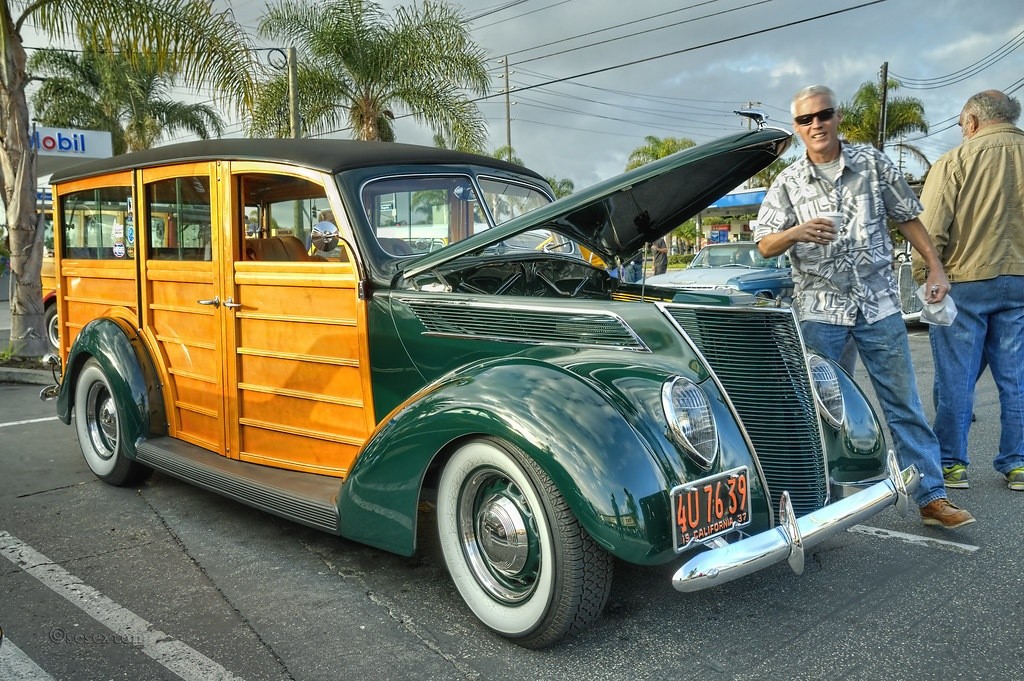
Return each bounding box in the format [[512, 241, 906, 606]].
[[338, 237, 414, 262], [246, 236, 328, 262]]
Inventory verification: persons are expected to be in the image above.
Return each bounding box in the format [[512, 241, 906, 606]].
[[623, 251, 642, 284], [751, 85, 976, 528], [249, 217, 258, 230], [313, 208, 341, 257], [651, 239, 668, 275], [912, 91, 1024, 493]]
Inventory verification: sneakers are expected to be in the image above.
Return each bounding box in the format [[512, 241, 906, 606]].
[[1002, 465, 1024, 490], [941, 465, 970, 488], [919, 496, 975, 527]]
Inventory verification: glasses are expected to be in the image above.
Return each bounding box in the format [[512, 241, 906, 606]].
[[795, 108, 836, 125]]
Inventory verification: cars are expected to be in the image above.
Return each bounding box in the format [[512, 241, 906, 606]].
[[635, 241, 795, 306], [36, 107, 926, 652], [34, 200, 212, 352]]
